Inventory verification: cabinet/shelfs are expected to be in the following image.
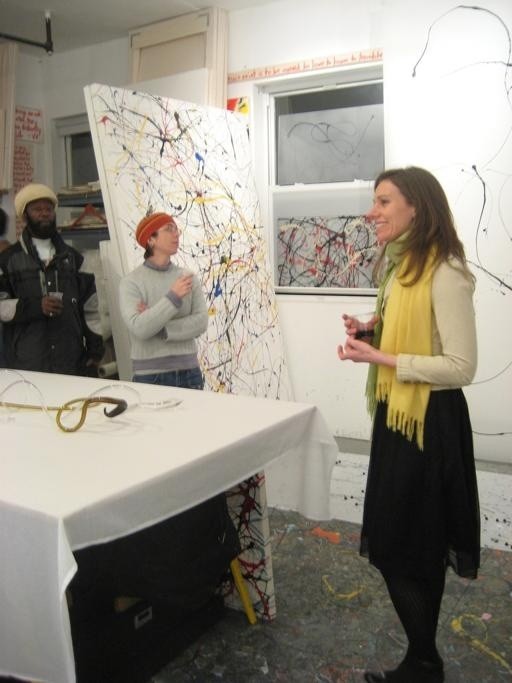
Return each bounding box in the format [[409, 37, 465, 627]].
[[56, 190, 109, 239]]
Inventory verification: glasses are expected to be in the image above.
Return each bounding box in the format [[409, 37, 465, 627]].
[[162, 223, 182, 236]]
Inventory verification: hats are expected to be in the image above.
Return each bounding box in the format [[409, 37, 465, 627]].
[[14, 183, 59, 217], [135, 212, 176, 248]]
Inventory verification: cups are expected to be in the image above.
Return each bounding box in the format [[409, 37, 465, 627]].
[[48, 291, 63, 318], [349, 310, 375, 346]]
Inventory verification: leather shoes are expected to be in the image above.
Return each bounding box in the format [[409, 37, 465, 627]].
[[364, 653, 445, 682]]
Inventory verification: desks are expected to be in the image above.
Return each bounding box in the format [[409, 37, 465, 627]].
[[0, 365, 319, 683]]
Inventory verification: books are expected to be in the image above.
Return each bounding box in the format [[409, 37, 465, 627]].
[[56, 179, 110, 230]]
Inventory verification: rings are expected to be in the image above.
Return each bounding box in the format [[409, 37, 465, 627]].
[[49, 311, 53, 317]]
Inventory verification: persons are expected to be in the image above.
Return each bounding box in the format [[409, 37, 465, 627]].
[[335, 165, 482, 682], [117, 212, 209, 393], [0, 183, 108, 378], [0, 208, 12, 252]]
